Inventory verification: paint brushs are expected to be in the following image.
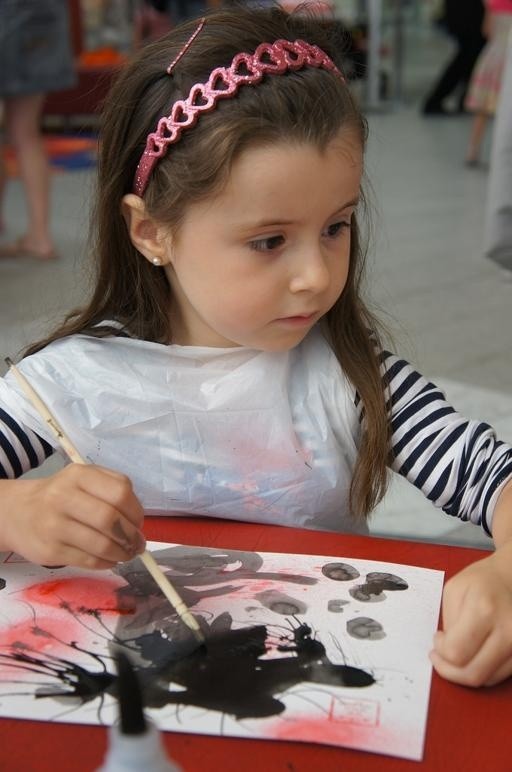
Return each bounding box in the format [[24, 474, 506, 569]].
[[0, 356, 227, 643]]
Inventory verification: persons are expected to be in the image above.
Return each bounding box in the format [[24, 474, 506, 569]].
[[422, 1, 511, 270], [0, 0, 512, 691], [0, 1, 80, 263]]
